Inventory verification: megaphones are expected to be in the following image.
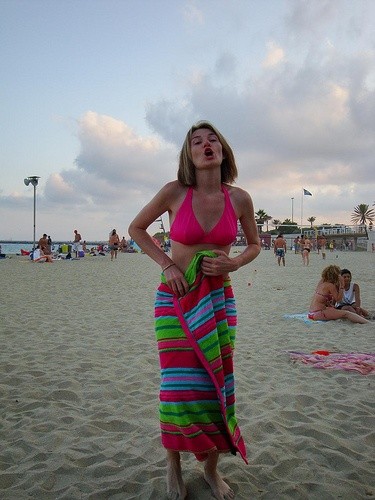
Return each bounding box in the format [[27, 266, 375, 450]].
[[31, 179, 38, 186], [25, 179, 31, 186]]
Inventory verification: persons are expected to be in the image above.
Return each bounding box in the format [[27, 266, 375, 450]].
[[334, 269, 369, 319], [319, 234, 327, 259], [300, 236, 311, 265], [307, 265, 368, 324], [83, 234, 353, 255], [274, 236, 287, 265], [299, 235, 305, 254], [47, 235, 52, 254], [110, 229, 120, 261], [73, 230, 82, 258], [128, 120, 261, 500], [38, 234, 50, 255]]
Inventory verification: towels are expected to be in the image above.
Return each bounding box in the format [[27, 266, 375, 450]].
[[284, 313, 330, 326], [153, 250, 249, 465], [286, 350, 375, 375]]
